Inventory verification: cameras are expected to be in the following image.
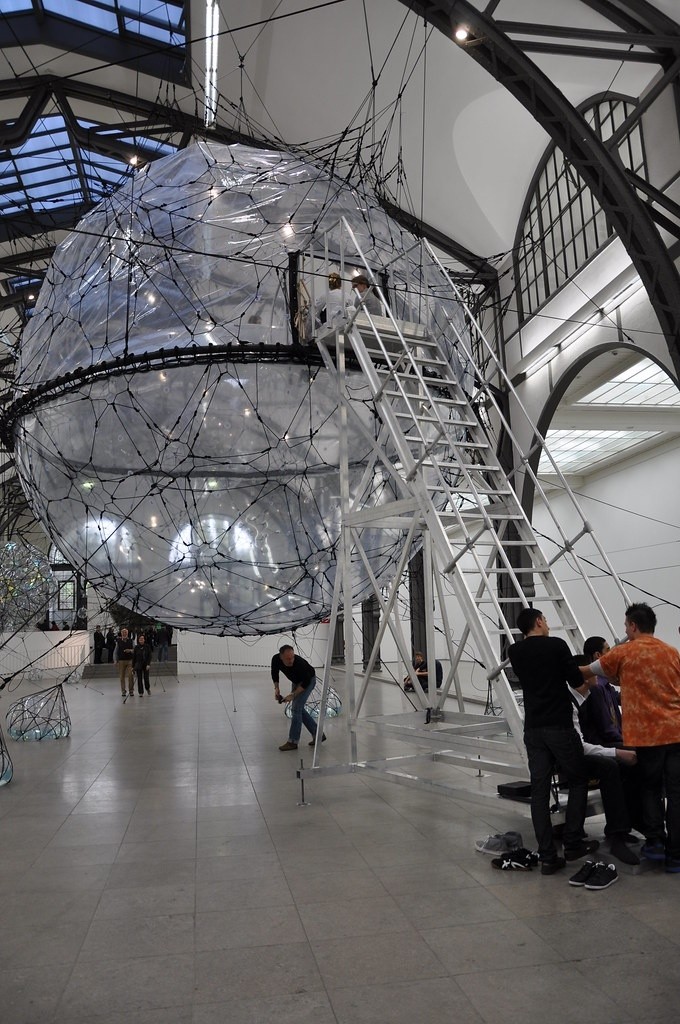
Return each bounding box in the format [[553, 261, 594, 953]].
[[278, 695, 285, 704]]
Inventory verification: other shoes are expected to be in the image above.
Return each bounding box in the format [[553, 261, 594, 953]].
[[122, 693, 126, 696], [541, 856, 566, 875], [407, 688, 415, 692], [564, 839, 600, 861], [95, 661, 104, 664], [147, 687, 151, 695], [130, 693, 134, 696], [139, 693, 143, 697], [309, 732, 326, 745], [108, 660, 114, 663], [278, 742, 298, 751]]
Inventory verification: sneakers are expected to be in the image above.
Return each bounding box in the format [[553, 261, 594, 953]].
[[491, 846, 540, 872], [664, 855, 680, 874], [640, 839, 666, 861], [584, 863, 619, 890], [568, 860, 606, 887], [474, 830, 524, 857]]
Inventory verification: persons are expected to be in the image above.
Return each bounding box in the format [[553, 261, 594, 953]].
[[508, 608, 599, 875], [72, 619, 87, 629], [94, 625, 105, 664], [106, 627, 116, 663], [113, 629, 134, 696], [271, 645, 327, 751], [404, 652, 443, 692], [573, 636, 642, 866], [51, 621, 59, 631], [62, 622, 69, 630], [578, 603, 680, 872], [36, 619, 50, 631], [132, 634, 152, 697], [351, 275, 382, 316], [307, 273, 353, 325], [157, 627, 168, 662]]
